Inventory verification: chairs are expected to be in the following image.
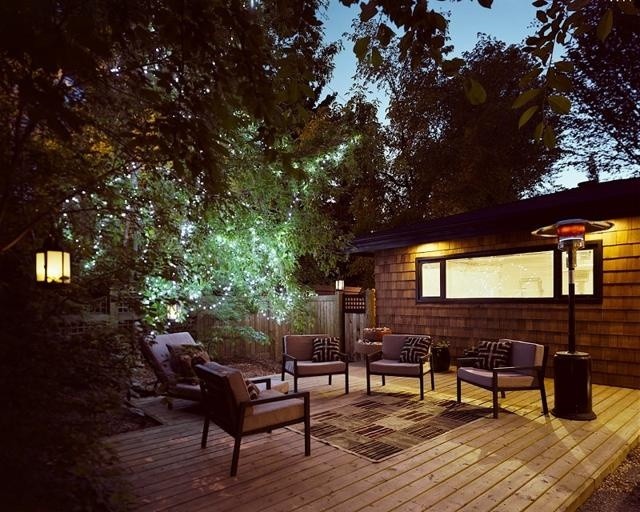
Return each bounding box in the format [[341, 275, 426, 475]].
[[138, 331, 271, 416], [282, 334, 349, 395], [190, 356, 310, 477], [457, 338, 550, 419], [366, 333, 434, 400]]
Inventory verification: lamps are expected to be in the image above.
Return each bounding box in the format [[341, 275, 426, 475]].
[[34, 228, 72, 289], [335, 272, 345, 291]]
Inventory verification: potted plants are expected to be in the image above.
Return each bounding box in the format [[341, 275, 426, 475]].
[[431, 339, 451, 373]]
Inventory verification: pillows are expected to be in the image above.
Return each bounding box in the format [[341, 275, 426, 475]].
[[245, 380, 260, 400], [472, 340, 513, 371], [399, 336, 433, 364], [166, 339, 211, 386], [312, 336, 346, 362]]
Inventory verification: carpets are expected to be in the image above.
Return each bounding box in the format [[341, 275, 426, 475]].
[[283, 389, 502, 463]]
